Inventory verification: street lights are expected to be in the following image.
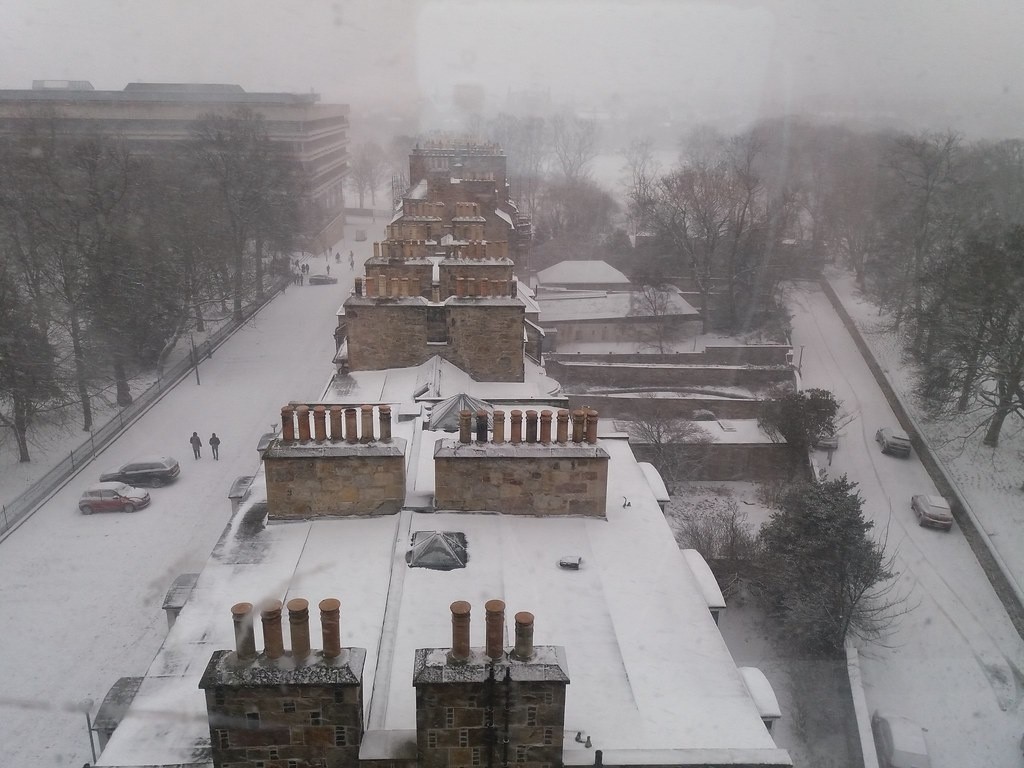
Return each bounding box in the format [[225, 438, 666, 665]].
[[188, 329, 200, 385]]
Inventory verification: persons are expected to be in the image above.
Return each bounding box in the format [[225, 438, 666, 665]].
[[293, 245, 354, 286], [190, 432, 202, 459], [209, 433, 220, 460]]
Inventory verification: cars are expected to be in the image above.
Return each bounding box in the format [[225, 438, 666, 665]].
[[309, 275, 337, 285], [811, 421, 838, 447]]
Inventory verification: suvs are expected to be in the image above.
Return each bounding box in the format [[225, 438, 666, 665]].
[[79, 482, 150, 514], [912, 494, 953, 528], [99, 455, 180, 488], [875, 426, 911, 458]]
